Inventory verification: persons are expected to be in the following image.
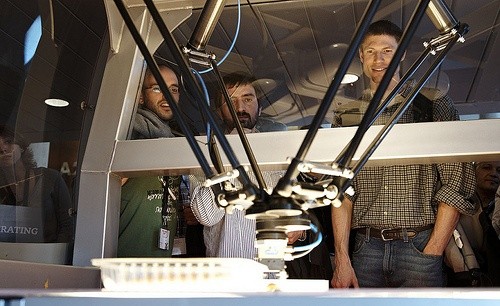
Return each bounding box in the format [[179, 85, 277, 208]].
[[118, 18, 500, 288], [0, 125, 76, 243]]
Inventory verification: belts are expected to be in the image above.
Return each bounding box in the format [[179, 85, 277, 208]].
[[357, 224, 433, 241]]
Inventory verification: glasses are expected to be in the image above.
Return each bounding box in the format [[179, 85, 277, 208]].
[[145, 85, 180, 95]]
